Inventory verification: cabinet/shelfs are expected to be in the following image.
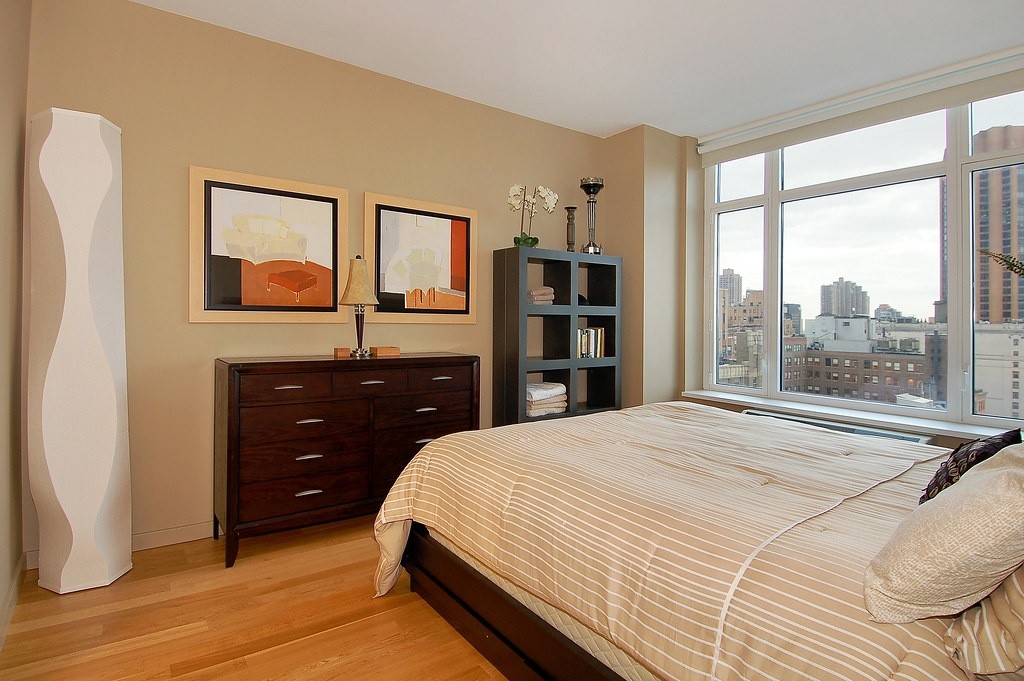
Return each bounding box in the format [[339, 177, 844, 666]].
[[492, 246, 623, 427], [213, 350, 481, 568]]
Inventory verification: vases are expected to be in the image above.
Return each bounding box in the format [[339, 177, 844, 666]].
[[514, 232, 539, 247]]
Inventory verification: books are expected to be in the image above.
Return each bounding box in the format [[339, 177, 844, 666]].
[[577, 327, 605, 359]]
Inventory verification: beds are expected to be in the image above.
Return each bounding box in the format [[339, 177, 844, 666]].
[[372, 400, 1024, 681]]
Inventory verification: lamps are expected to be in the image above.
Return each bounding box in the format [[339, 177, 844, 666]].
[[339, 254, 381, 357]]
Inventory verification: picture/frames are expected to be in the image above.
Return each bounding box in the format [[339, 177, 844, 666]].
[[188, 165, 350, 324], [363, 191, 477, 324]]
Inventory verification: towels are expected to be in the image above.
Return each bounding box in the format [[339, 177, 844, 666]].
[[526, 381, 567, 417], [527, 286, 554, 303]]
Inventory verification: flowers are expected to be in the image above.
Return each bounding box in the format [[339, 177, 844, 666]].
[[507, 182, 559, 236]]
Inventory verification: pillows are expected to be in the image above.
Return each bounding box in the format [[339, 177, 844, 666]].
[[862, 442, 1024, 623], [919, 426, 1023, 505]]
